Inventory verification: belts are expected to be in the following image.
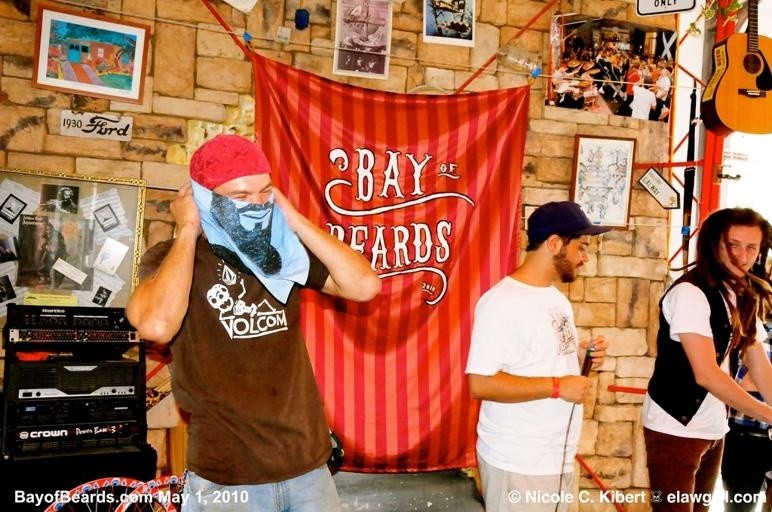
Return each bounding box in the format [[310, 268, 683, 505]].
[[728, 420, 772, 441]]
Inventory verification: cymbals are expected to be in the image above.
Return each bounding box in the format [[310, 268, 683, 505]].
[[568, 59, 582, 67], [584, 61, 594, 70]]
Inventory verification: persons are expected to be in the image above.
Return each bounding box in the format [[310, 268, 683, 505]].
[[124, 134, 383, 510], [721, 303, 772, 512], [46, 188, 77, 212], [641, 207, 771, 512], [466, 200, 609, 511], [39, 224, 65, 289], [554, 31, 673, 124]]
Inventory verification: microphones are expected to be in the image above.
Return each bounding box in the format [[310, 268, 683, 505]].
[[577, 338, 601, 377]]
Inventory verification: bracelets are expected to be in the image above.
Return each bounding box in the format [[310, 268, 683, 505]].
[[552, 377, 560, 399]]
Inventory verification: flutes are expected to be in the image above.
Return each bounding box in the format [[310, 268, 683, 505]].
[[39, 200, 66, 206]]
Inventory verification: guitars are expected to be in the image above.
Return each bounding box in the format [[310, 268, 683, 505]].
[[701, 1, 771, 134]]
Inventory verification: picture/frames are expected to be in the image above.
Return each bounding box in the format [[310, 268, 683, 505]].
[[569, 134, 636, 229], [0, 166, 147, 333], [31, 2, 150, 105]]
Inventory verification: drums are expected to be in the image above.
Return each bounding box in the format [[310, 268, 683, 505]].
[[582, 85, 599, 106]]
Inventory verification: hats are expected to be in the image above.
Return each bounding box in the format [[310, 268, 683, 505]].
[[190, 134, 272, 191], [526, 200, 614, 244]]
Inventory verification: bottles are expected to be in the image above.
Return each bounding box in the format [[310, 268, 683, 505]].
[[496, 43, 541, 79]]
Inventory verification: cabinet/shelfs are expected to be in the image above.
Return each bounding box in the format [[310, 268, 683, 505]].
[[1, 304, 157, 512]]
